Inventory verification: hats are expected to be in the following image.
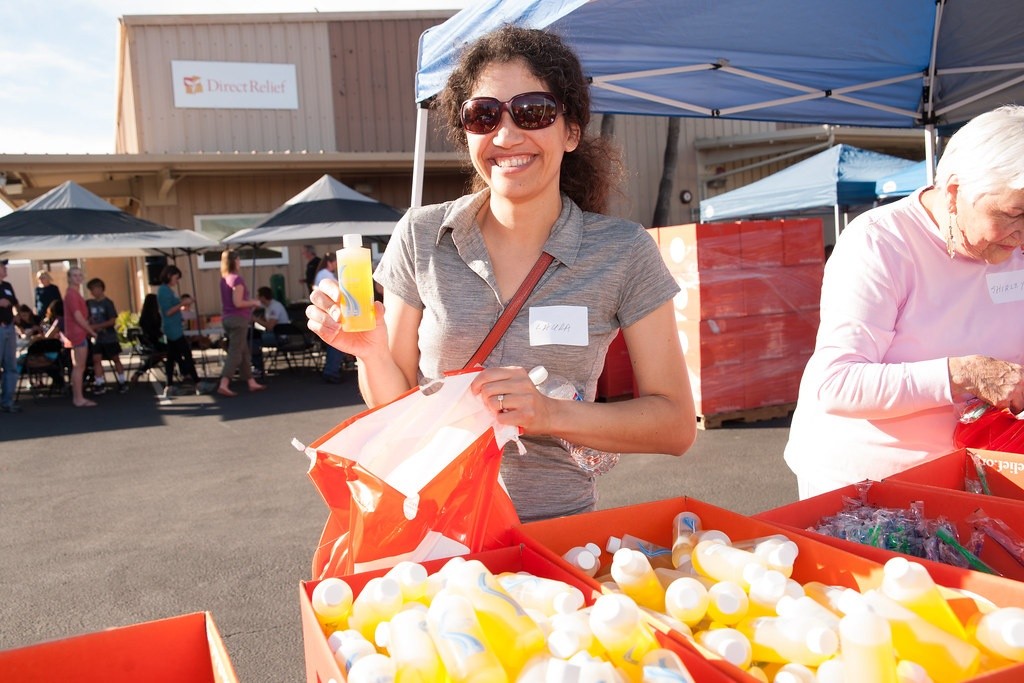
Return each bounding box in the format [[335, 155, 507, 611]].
[[0, 259, 8, 265]]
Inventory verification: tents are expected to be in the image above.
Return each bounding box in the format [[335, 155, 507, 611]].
[[409, 1, 1024, 248], [222, 174, 407, 353], [0, 180, 221, 380]]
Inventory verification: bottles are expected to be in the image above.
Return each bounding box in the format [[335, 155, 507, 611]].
[[606, 534, 1024, 683], [671, 511, 700, 568], [312, 554, 696, 683], [560, 542, 601, 577], [335, 233, 375, 332], [528, 365, 620, 476]]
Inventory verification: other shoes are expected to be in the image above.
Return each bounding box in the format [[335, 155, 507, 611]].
[[217, 388, 239, 396], [249, 384, 268, 390], [119, 384, 130, 394], [94, 382, 107, 395]]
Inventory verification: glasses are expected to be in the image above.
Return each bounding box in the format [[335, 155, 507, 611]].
[[458, 90, 567, 136]]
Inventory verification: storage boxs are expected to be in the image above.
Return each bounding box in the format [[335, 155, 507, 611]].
[[1, 446, 1024, 682], [640, 216, 825, 415]]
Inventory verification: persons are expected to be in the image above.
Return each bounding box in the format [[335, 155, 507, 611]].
[[305, 25, 695, 521], [0, 245, 384, 412], [782, 104, 1024, 500]]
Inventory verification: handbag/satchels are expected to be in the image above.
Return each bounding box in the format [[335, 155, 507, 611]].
[[305, 364, 522, 581]]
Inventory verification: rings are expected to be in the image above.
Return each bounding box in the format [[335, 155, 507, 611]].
[[497, 395, 505, 409]]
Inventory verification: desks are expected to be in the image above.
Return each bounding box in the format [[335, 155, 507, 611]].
[[184, 328, 226, 385]]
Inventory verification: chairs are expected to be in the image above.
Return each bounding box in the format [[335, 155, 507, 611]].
[[14, 303, 357, 403]]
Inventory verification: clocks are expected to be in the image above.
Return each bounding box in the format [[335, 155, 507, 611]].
[[681, 191, 692, 202]]
